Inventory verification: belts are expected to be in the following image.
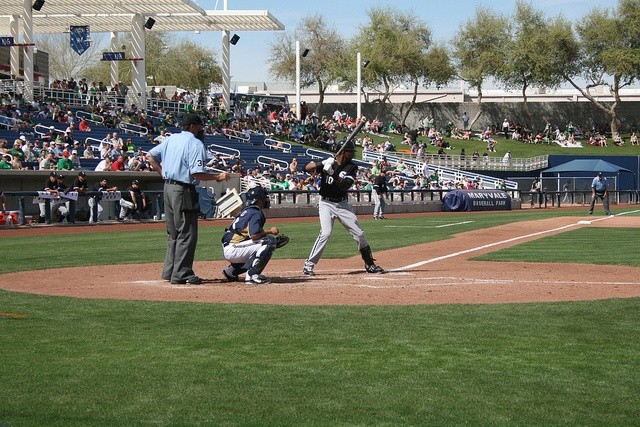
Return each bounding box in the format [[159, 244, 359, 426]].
[[322, 195, 347, 203]]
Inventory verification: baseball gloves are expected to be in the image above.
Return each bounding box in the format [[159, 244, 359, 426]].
[[275, 233, 289, 248]]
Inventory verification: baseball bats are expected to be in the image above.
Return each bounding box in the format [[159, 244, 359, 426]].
[[323, 119, 366, 172]]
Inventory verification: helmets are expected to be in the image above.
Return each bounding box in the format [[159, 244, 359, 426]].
[[247, 183, 271, 208], [336, 140, 357, 152]]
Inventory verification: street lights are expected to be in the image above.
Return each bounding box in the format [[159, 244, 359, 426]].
[[222, 31, 240, 113], [295, 40, 310, 121], [22, 0, 47, 106], [356, 52, 370, 120], [131, 14, 156, 110]]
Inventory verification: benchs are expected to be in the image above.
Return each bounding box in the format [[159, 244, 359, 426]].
[[1, 103, 400, 179]]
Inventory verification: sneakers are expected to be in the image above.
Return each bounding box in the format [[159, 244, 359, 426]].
[[171, 275, 201, 284], [245, 271, 271, 284], [303, 268, 314, 276], [379, 216, 386, 220], [223, 269, 238, 280], [366, 264, 383, 273]]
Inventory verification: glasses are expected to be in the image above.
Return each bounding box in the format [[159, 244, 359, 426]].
[[30, 145, 34, 148]]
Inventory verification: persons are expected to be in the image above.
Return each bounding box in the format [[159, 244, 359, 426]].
[[36, 172, 60, 223], [0, 188, 6, 213], [301, 140, 386, 277], [500, 115, 609, 148], [205, 145, 381, 194], [612, 131, 625, 147], [630, 132, 639, 146], [371, 170, 390, 220], [530, 175, 543, 207], [588, 171, 614, 215], [88, 179, 119, 224], [381, 145, 513, 198], [220, 185, 289, 284], [563, 182, 571, 203], [145, 112, 231, 285], [119, 179, 145, 222], [0, 78, 156, 136], [64, 171, 88, 221], [0, 136, 156, 172]]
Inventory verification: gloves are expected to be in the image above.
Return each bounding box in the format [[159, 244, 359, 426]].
[[323, 162, 334, 176], [321, 157, 335, 165]]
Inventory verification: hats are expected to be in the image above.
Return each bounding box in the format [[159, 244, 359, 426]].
[[50, 172, 58, 177], [14, 139, 21, 145], [182, 113, 202, 125], [598, 172, 603, 175], [79, 172, 87, 176], [20, 135, 26, 141], [55, 140, 62, 146], [72, 150, 77, 155], [535, 177, 537, 179], [262, 171, 269, 175], [74, 141, 79, 145], [137, 146, 142, 149], [132, 179, 139, 183], [50, 141, 55, 145], [64, 151, 69, 157]]
